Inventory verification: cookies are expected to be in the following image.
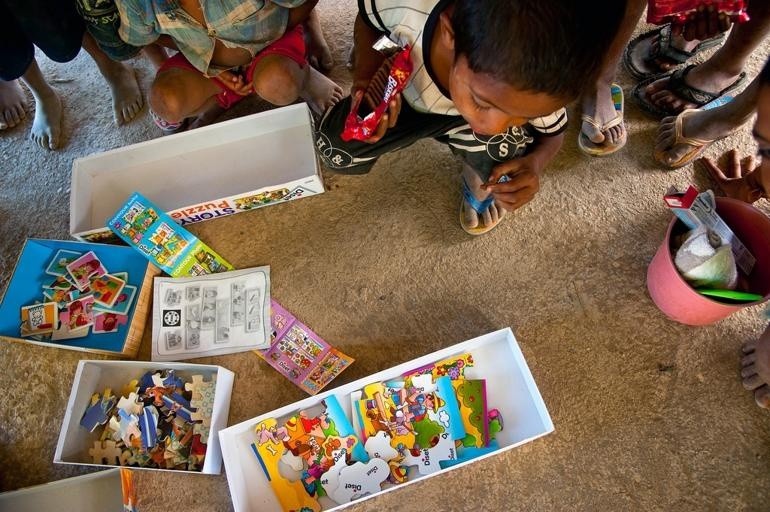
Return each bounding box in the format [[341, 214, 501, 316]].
[[362, 47, 398, 110]]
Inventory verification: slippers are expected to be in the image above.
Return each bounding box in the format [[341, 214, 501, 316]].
[[577, 85, 626, 156], [630, 71, 747, 112], [458, 175, 504, 236], [661, 106, 714, 168], [620, 17, 726, 77]]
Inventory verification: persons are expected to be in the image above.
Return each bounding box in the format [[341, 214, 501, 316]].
[[314, 1, 627, 235], [0, 76, 29, 135], [0, 0, 148, 152], [113, 0, 345, 136], [575, 0, 768, 415]]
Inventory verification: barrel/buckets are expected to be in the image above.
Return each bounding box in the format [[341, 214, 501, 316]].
[[647, 197, 770, 326]]
